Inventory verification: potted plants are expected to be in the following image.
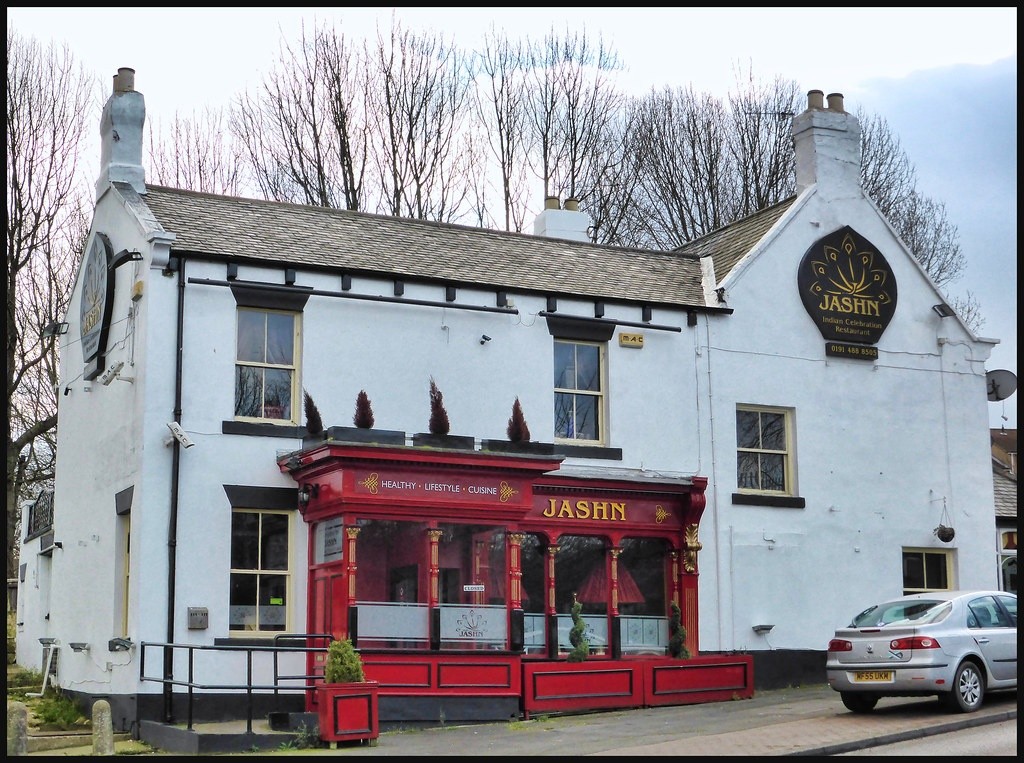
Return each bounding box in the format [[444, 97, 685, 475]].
[[327, 389, 405, 445], [411, 374, 475, 450], [481, 394, 555, 455], [302, 387, 328, 449], [316, 636, 379, 749], [933, 524, 955, 542]]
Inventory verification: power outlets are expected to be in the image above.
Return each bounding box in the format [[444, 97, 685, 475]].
[[938, 336, 947, 346], [695, 347, 702, 358], [106, 662, 112, 671]]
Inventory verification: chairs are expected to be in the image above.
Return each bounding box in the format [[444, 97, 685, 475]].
[[971, 606, 999, 628]]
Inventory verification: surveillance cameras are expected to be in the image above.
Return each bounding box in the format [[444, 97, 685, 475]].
[[97, 361, 125, 387], [482, 335, 491, 341], [166, 421, 195, 449]]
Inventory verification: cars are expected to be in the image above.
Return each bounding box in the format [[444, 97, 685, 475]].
[[826, 590, 1015, 713]]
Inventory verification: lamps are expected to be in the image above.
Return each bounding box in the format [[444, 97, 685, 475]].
[[1001, 400, 1008, 421], [1000, 424, 1007, 436]]
